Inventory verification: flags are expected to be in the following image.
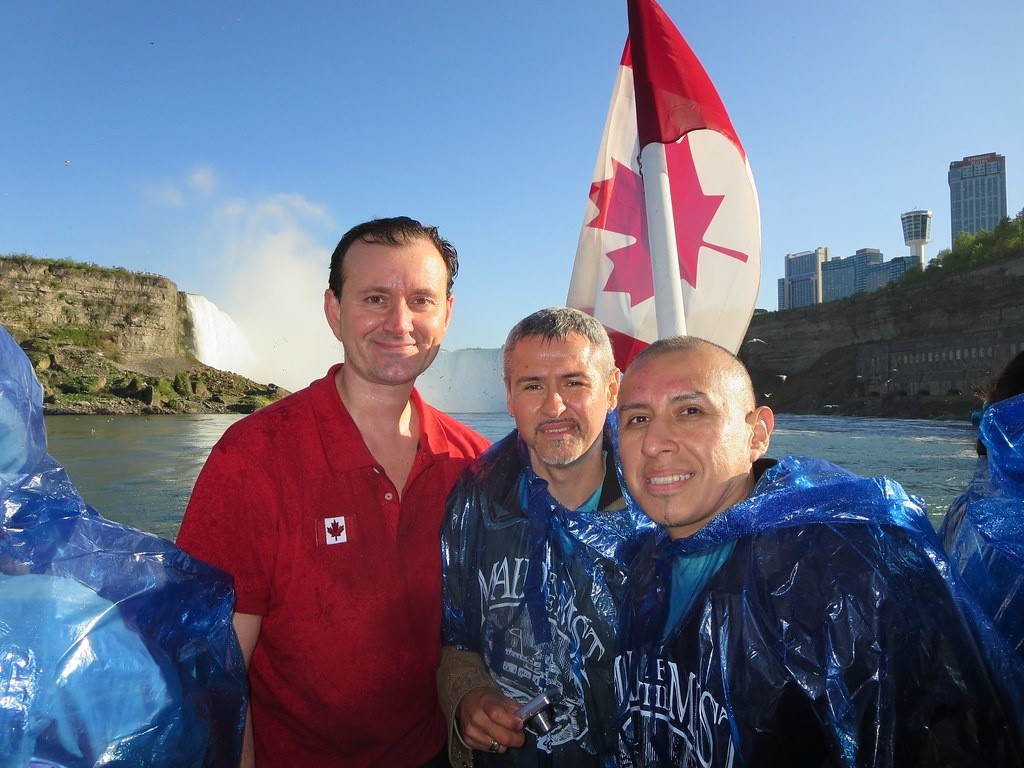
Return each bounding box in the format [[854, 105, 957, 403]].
[[565, 0, 763, 374]]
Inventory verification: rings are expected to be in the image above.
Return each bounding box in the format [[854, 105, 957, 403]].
[[488, 740, 500, 752]]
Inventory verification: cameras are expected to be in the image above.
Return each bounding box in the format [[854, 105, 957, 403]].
[[512, 690, 565, 736]]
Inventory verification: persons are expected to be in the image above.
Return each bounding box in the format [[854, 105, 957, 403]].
[[177, 216, 493, 768], [938, 351, 1024, 670], [0, 324, 250, 768], [436, 306, 1024, 768]]
